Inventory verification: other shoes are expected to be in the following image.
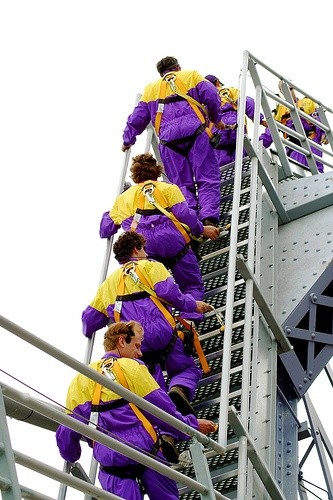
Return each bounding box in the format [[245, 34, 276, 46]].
[[204, 220, 223, 231], [169, 386, 197, 418], [162, 435, 179, 464]]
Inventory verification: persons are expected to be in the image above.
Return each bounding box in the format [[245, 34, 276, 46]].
[[55, 319, 217, 500], [121, 56, 224, 228], [204, 74, 268, 167], [100, 153, 220, 328], [82, 231, 212, 464], [259, 79, 329, 174]]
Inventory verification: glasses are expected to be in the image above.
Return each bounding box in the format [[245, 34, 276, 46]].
[[290, 88, 293, 90]]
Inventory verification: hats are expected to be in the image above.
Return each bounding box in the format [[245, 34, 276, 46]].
[[205, 75, 224, 86]]
